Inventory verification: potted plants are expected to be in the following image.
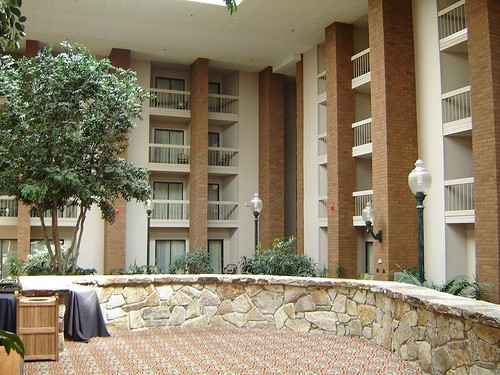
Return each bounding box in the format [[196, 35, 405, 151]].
[[0, 330, 25, 375]]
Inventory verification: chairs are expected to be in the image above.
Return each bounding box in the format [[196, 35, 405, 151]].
[[178, 153, 188, 164], [218, 154, 229, 166]]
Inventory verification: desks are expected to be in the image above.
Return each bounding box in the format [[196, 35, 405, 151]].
[[0, 294, 16, 333]]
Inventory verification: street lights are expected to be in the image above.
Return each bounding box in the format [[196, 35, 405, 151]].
[[144, 191, 155, 274], [250, 192, 263, 276], [407, 158, 432, 290]]
